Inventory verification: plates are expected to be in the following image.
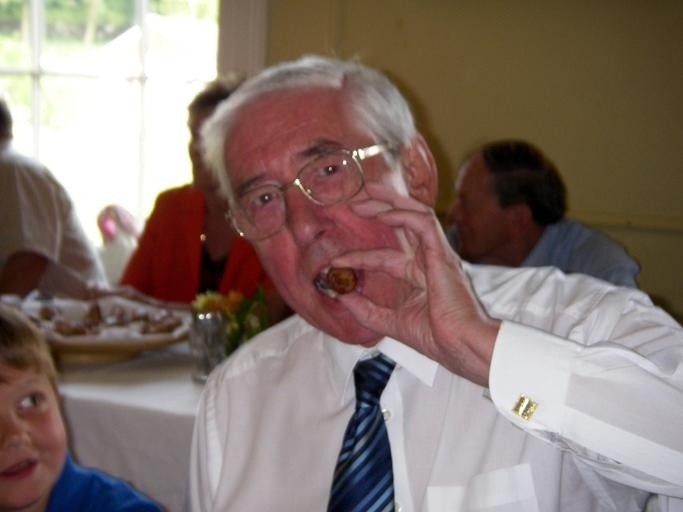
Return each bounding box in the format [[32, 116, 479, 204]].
[[18, 291, 192, 367]]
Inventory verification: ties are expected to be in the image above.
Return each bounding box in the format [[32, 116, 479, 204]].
[[328, 353, 398, 512]]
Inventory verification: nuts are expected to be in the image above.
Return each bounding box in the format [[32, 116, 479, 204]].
[[327, 267, 356, 293]]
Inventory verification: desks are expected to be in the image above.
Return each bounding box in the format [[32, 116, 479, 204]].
[[56, 341, 206, 512]]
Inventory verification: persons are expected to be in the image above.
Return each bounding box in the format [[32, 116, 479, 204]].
[[1, 301, 167, 512], [445, 137, 643, 289], [1, 95, 112, 297], [80, 71, 286, 328], [184, 52, 683, 511]]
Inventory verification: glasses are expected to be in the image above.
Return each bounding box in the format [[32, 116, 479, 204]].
[[225, 141, 391, 244]]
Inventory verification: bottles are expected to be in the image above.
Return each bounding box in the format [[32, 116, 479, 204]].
[[188, 312, 226, 387]]
